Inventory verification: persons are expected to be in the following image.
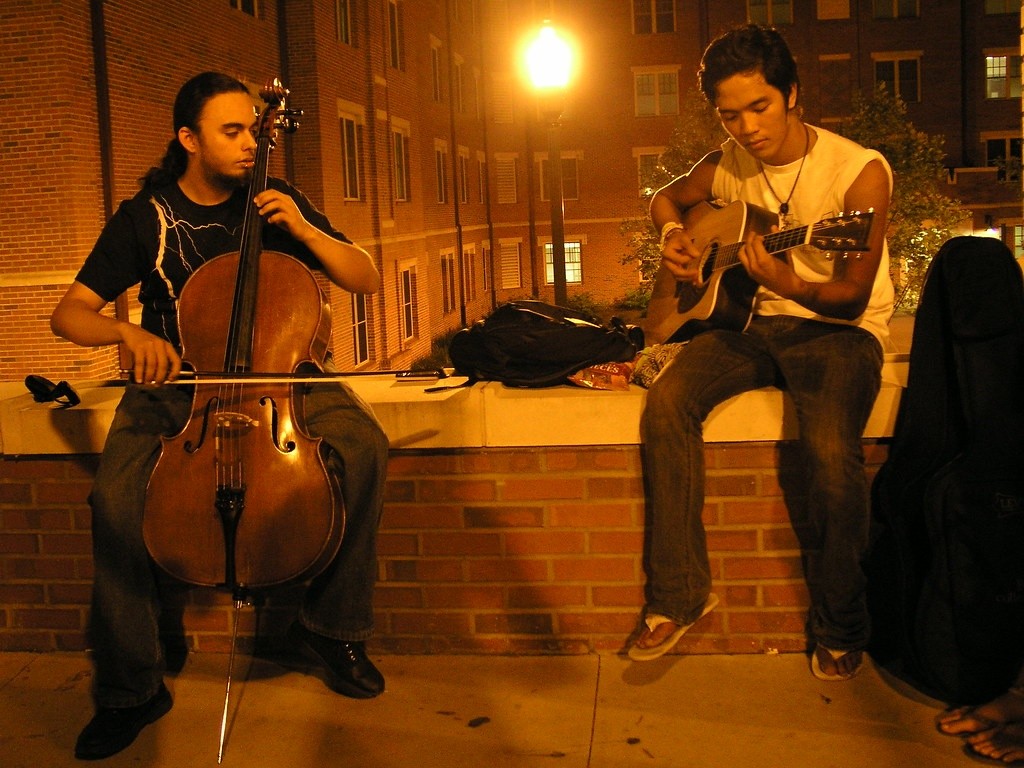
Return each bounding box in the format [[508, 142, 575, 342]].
[[939, 673, 1024, 763], [51, 69, 389, 762], [627, 23, 894, 681]]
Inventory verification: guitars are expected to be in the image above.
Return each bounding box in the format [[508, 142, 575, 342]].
[[640, 194, 882, 350]]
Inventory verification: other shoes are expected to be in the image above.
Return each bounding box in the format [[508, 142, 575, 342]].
[[72, 679, 173, 760], [293, 627, 384, 699]]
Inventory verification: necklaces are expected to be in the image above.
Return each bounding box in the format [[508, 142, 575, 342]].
[[759, 120, 809, 213]]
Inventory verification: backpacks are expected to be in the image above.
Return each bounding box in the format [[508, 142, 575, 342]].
[[448, 300, 645, 388]]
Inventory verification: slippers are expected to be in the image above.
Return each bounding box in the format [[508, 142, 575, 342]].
[[937, 703, 1001, 738], [812, 641, 867, 681], [628, 593, 719, 662], [961, 721, 1024, 768]]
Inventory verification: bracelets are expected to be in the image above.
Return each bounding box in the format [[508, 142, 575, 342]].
[[658, 221, 683, 250]]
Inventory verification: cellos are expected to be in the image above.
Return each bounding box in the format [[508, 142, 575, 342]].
[[134, 78, 349, 765]]
